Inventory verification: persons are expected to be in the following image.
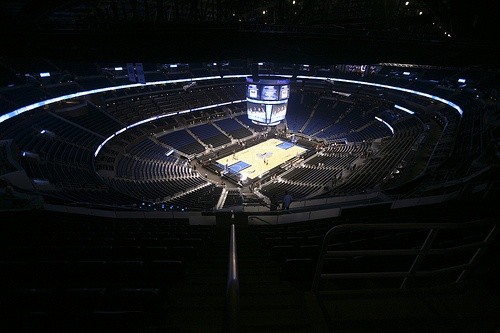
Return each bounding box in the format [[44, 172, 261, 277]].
[[269, 194, 279, 215], [191, 124, 379, 194], [281, 190, 292, 214]]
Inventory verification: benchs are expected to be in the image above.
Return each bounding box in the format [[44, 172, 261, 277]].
[[0, 62, 500, 332]]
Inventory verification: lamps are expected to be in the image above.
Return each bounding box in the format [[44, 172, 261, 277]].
[[228, 0, 453, 40]]
